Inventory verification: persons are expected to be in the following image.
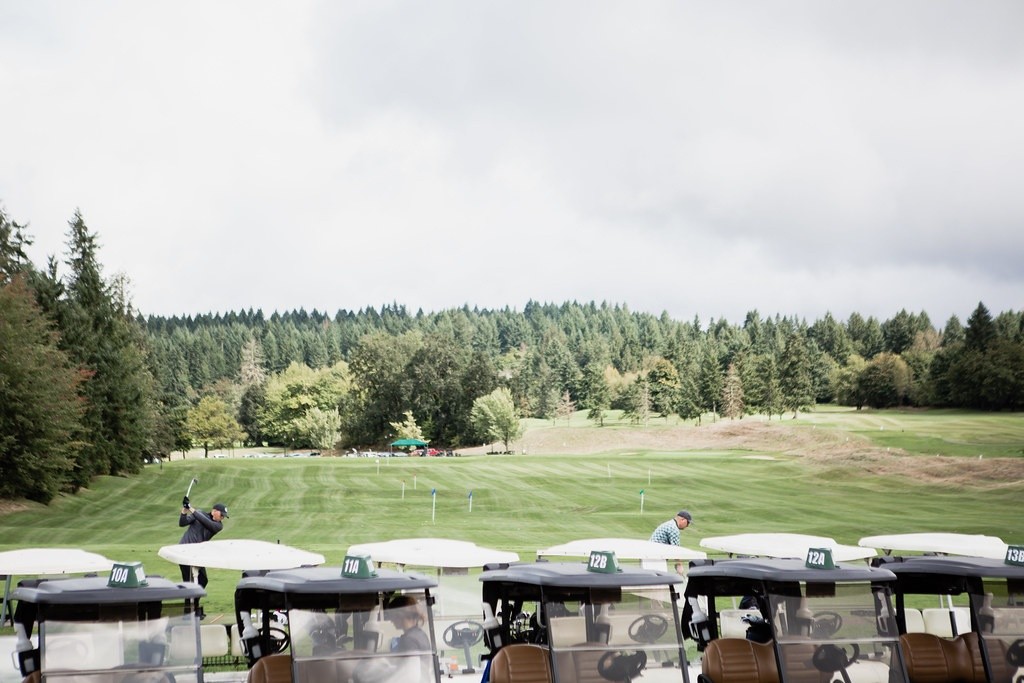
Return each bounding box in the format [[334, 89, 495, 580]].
[[642, 511, 691, 621], [386, 598, 431, 682], [178, 496, 229, 602]]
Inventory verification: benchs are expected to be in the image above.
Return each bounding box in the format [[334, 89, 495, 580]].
[[697, 638, 834, 683], [22, 664, 175, 683], [490, 643, 621, 683], [889, 633, 1018, 683], [246, 649, 373, 683]]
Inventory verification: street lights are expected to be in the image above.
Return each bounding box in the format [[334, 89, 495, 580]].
[[284, 436, 295, 457]]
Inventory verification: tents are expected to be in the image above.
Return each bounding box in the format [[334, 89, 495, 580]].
[[389, 440, 428, 457]]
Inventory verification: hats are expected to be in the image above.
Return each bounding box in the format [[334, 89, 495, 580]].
[[213, 503, 229, 519], [678, 511, 695, 524]]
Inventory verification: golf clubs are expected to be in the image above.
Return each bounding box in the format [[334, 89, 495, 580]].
[[186, 477, 199, 498], [684, 572, 706, 610]]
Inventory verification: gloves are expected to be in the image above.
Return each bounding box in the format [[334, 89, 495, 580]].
[[183, 496, 190, 510]]
